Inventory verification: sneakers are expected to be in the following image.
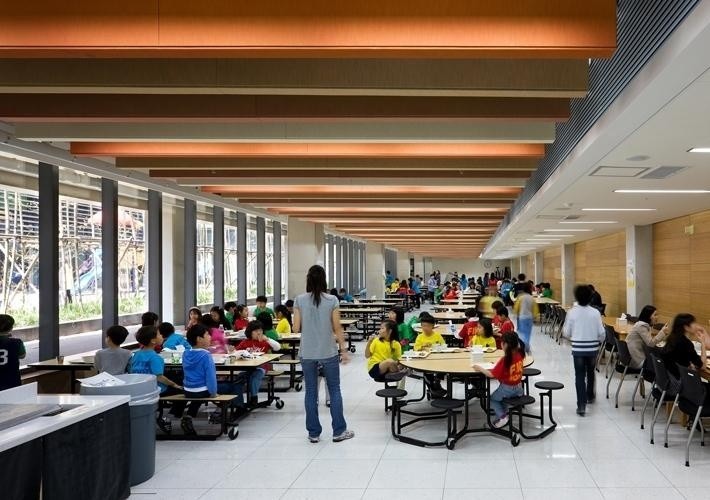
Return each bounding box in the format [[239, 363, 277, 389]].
[[577, 408, 585, 413], [156, 417, 172, 432], [208, 413, 221, 424], [333, 431, 354, 442], [308, 436, 319, 443], [495, 416, 509, 428], [385, 372, 402, 380], [181, 416, 197, 435]]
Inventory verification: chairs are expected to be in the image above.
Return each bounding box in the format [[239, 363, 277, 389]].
[[541, 302, 710, 467]]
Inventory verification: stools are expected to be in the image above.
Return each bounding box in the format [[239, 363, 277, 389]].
[[375, 290, 565, 451]]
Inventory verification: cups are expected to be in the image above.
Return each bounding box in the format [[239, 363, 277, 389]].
[[431, 344, 449, 352], [402, 347, 414, 364], [56, 356, 64, 364]]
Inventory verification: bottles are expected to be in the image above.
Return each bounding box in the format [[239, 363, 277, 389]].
[[218, 324, 225, 338], [470, 329, 483, 368]]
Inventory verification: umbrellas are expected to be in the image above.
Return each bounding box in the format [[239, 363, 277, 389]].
[[85, 206, 133, 229]]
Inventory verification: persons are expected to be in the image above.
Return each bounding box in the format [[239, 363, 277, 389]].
[[366, 267, 554, 431], [330, 287, 359, 305], [626, 303, 710, 417], [59, 262, 73, 308], [0, 315, 27, 389], [93, 294, 296, 436], [563, 283, 605, 417], [291, 264, 355, 445]]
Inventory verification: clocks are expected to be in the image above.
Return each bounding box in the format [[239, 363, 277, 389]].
[[484, 259, 491, 268]]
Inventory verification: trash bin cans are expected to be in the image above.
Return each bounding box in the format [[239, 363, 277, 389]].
[[79, 373, 162, 486]]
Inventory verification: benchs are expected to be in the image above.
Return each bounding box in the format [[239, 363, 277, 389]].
[[26, 288, 428, 500]]
